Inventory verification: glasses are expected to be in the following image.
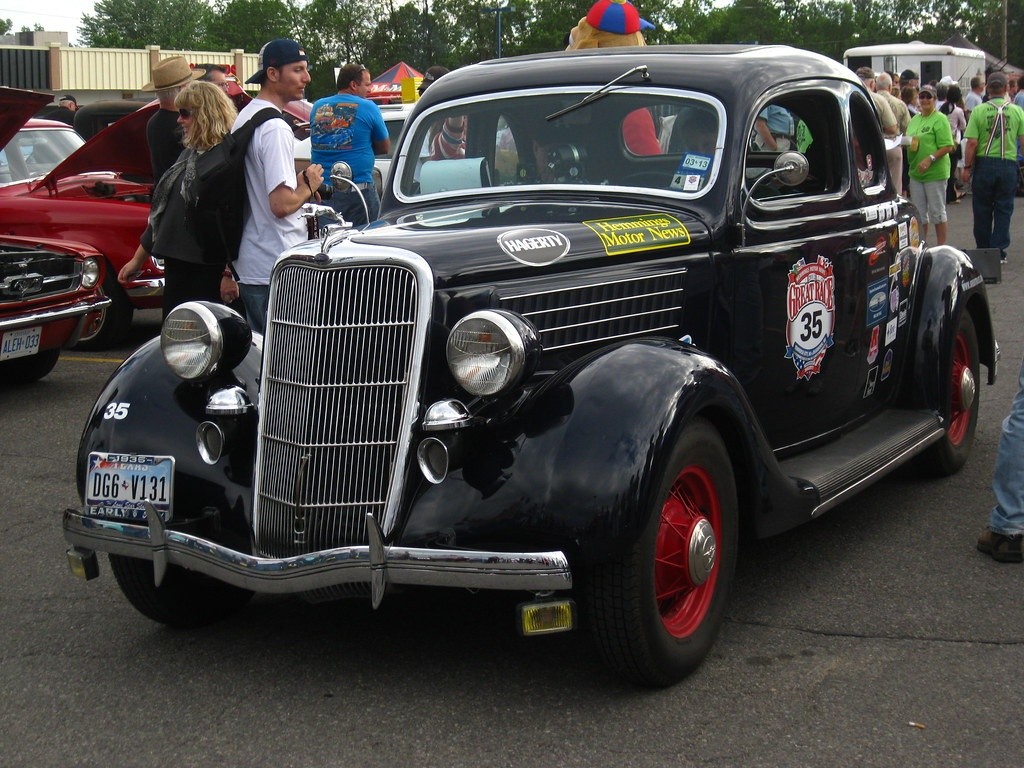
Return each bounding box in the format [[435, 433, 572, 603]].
[[357, 64, 366, 72], [178, 109, 192, 118], [216, 83, 230, 89]]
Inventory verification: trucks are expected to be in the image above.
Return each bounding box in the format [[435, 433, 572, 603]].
[[843, 43, 986, 101]]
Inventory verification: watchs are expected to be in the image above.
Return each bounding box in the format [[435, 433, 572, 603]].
[[930, 155, 936, 162]]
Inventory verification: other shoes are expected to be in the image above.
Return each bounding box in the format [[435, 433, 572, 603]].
[[977, 531, 1024, 564]]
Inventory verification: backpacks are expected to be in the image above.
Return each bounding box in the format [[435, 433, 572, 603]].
[[182, 108, 289, 282]]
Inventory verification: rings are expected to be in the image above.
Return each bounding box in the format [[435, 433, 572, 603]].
[[229, 300, 233, 302], [925, 166, 927, 168]]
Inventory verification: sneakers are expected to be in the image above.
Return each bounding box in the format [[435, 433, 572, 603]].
[[994, 250, 1008, 263]]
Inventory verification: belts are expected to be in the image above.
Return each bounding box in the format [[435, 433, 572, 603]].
[[770, 132, 792, 139]]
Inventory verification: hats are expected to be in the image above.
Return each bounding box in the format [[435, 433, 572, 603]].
[[900, 70, 919, 80], [939, 76, 958, 86], [139, 56, 207, 93], [855, 67, 874, 79], [245, 38, 309, 85], [532, 104, 581, 147], [418, 66, 450, 90], [59, 95, 79, 108], [918, 84, 937, 97], [986, 71, 1009, 88]]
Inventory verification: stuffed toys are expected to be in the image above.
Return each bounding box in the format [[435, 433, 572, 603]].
[[561, 0, 661, 154]]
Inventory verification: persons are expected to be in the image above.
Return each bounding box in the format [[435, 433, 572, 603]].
[[661, 66, 1024, 262], [117, 38, 518, 330], [38, 94, 81, 163], [975, 362, 1024, 561]]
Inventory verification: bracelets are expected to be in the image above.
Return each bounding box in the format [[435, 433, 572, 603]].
[[222, 270, 233, 277]]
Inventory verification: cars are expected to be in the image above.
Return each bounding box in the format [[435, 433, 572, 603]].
[[283, 99, 432, 198], [61, 56, 1004, 686], [0, 84, 114, 381], [1, 97, 168, 348]]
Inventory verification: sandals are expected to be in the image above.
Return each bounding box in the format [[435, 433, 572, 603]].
[[955, 191, 966, 199]]
